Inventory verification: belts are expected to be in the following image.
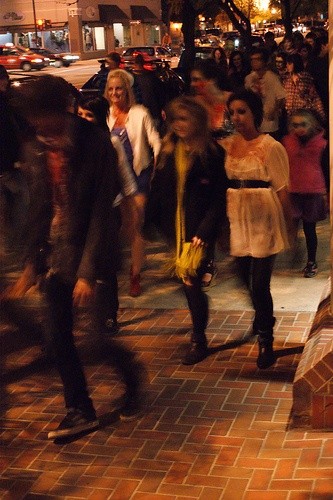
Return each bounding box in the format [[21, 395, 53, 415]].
[[228, 180, 271, 188]]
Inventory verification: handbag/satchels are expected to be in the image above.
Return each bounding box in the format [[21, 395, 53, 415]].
[[175, 242, 217, 287]]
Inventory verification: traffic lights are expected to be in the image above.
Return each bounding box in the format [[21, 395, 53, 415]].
[[38, 20, 43, 30]]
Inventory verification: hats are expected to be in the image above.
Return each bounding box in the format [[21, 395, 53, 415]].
[[227, 90, 263, 127], [107, 69, 134, 88]]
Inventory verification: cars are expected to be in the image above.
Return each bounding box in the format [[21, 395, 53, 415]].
[[0, 44, 78, 72], [194, 23, 306, 51], [119, 46, 180, 74]]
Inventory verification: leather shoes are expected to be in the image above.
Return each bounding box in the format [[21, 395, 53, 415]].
[[182, 339, 209, 365]]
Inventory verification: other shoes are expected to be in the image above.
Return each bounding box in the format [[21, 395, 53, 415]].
[[256, 346, 274, 369], [102, 316, 118, 337]]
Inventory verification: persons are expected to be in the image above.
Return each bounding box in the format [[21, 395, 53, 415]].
[[104, 69, 161, 297], [143, 96, 228, 366], [278, 108, 329, 278], [76, 94, 137, 336], [218, 91, 294, 368], [101, 28, 329, 233], [17, 73, 143, 437], [0, 64, 32, 260]]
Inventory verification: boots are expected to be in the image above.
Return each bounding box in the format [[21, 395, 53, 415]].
[[130, 267, 141, 298]]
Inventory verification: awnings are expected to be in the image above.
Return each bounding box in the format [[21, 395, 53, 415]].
[[98, 4, 130, 27], [130, 6, 156, 22]]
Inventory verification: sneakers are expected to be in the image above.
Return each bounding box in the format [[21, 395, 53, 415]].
[[48, 408, 99, 438], [120, 385, 146, 422], [303, 262, 318, 278]]
